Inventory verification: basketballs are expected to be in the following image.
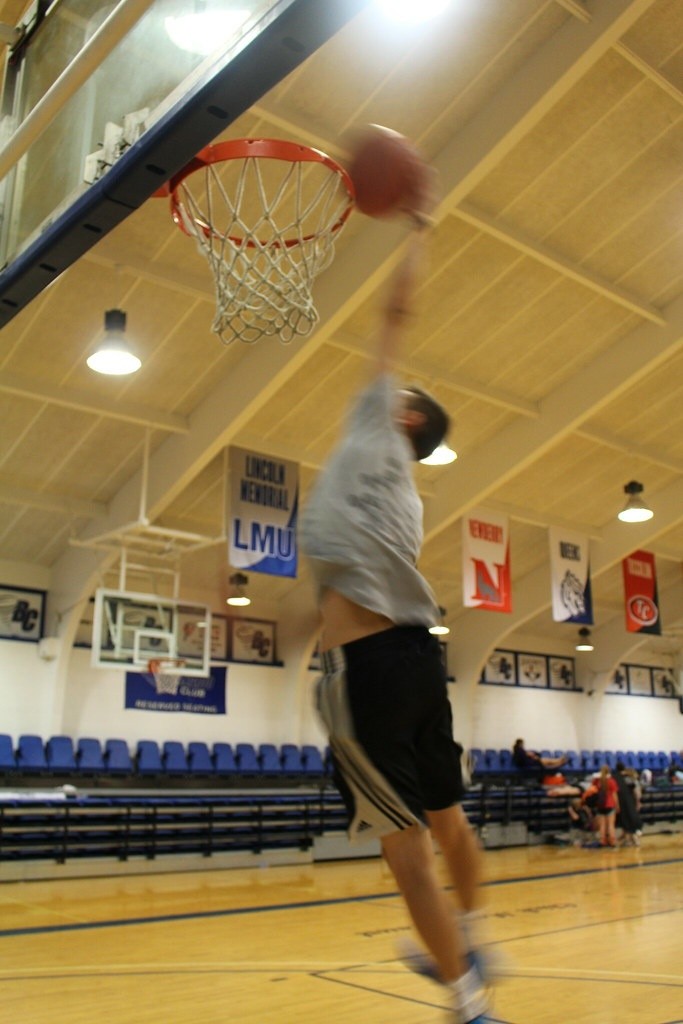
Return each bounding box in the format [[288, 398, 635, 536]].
[[340, 122, 426, 219]]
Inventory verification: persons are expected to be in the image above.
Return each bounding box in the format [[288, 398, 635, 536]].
[[511, 737, 682, 850], [287, 159, 513, 1022]]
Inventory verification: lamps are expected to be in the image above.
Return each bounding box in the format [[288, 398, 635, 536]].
[[575, 627, 594, 652], [88, 309, 142, 375], [429, 607, 451, 635], [617, 480, 654, 524], [226, 572, 251, 606]]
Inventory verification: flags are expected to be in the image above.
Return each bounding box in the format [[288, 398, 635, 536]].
[[622, 548, 664, 637], [224, 441, 303, 580], [460, 502, 514, 613], [548, 525, 596, 626]]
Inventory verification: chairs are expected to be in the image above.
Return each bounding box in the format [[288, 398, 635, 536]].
[[468, 748, 683, 776], [0, 735, 326, 777]]
